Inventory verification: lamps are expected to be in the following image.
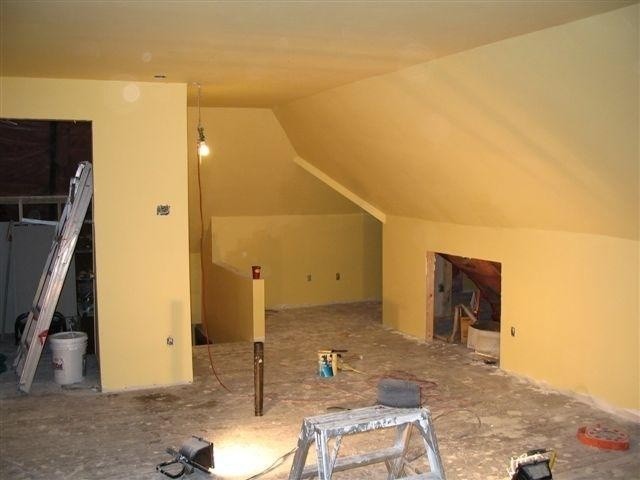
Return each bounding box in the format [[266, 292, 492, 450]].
[[156, 434, 216, 477]]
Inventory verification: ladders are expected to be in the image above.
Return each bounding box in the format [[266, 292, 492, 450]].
[[13, 161, 92, 393], [288, 405, 446, 480]]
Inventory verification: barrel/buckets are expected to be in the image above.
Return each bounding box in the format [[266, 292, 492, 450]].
[[49, 332, 89, 385], [317, 350, 338, 377]]
